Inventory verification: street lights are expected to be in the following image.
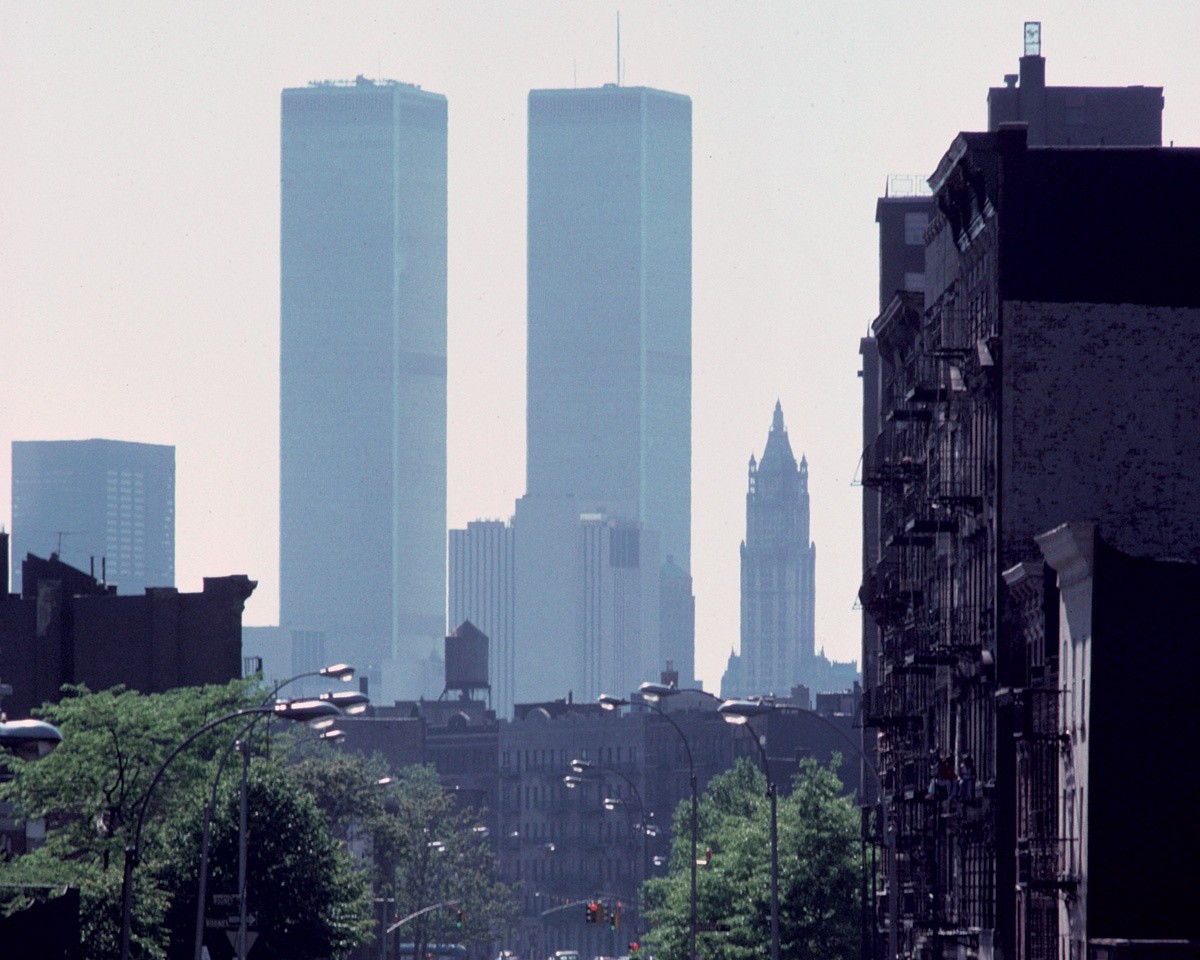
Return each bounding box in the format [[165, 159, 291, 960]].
[[564, 758, 667, 960], [717, 699, 900, 959], [413, 825, 492, 959], [637, 681, 783, 960], [120, 663, 372, 960], [595, 692, 699, 960], [327, 777, 395, 831]]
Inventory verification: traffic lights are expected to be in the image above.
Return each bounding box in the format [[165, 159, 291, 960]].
[[455, 909, 465, 929], [610, 912, 620, 933], [586, 900, 598, 923]]
